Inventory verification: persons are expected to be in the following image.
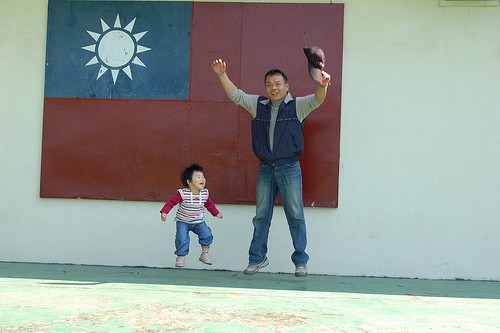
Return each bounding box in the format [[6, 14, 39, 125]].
[[211, 57, 331, 277], [160, 163, 225, 267]]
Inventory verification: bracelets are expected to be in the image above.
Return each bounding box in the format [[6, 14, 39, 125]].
[[320, 84, 327, 88]]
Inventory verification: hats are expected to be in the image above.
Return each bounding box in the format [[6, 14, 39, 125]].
[[304, 44, 325, 85]]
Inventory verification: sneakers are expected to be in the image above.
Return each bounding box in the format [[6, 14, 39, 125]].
[[199, 252, 213, 264], [244, 255, 269, 274], [175, 256, 186, 267], [295, 265, 308, 277]]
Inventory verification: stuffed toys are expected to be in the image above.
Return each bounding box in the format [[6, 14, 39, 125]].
[[304, 46, 326, 85]]
